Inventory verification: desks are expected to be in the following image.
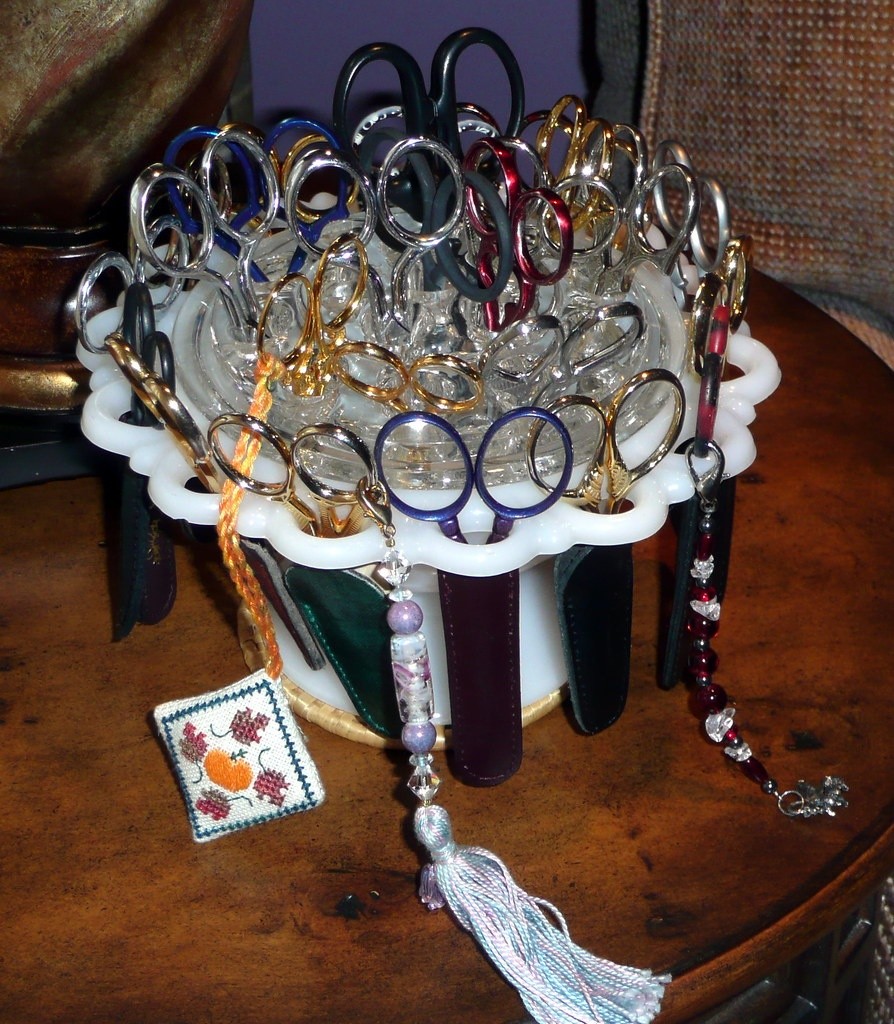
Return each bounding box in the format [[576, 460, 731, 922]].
[[1, 256, 894, 1024]]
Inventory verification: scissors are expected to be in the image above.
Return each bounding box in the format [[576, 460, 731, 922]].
[[66, 26, 751, 547]]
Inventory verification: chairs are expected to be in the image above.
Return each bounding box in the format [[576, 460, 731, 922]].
[[590, 0, 894, 376]]
